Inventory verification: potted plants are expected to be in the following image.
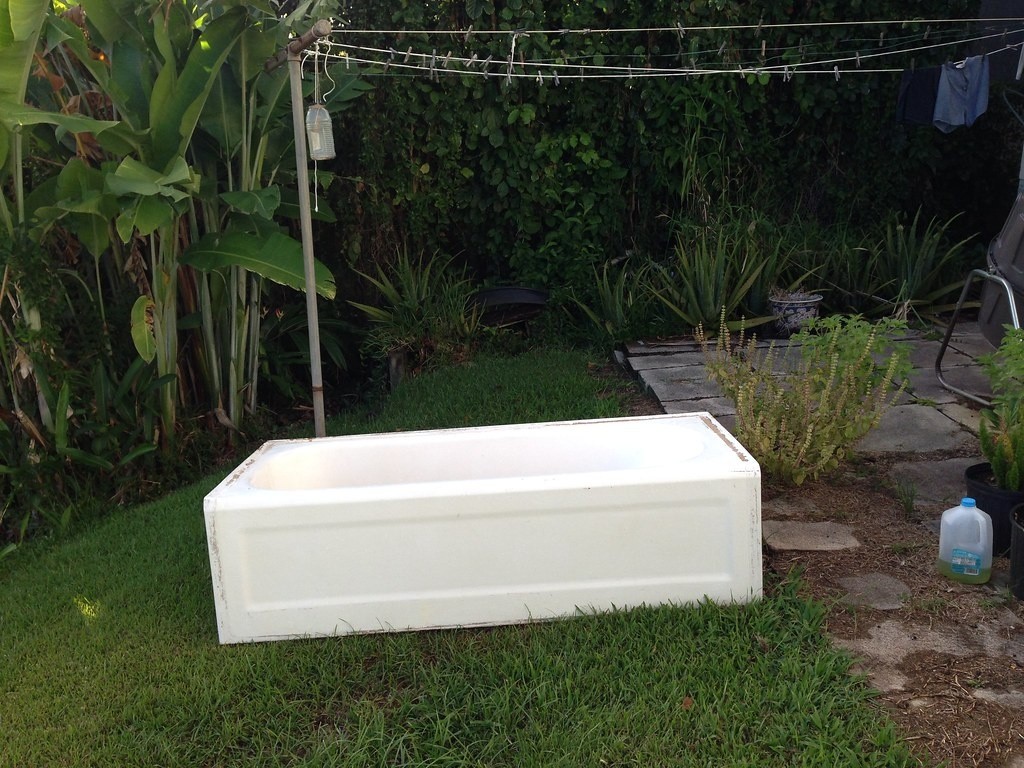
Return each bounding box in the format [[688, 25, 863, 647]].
[[964, 323, 1024, 602]]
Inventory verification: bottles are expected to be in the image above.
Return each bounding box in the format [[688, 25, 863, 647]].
[[938, 497, 993, 584], [306, 103, 335, 160]]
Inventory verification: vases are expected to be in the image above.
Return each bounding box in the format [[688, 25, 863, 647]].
[[770, 294, 823, 338]]
